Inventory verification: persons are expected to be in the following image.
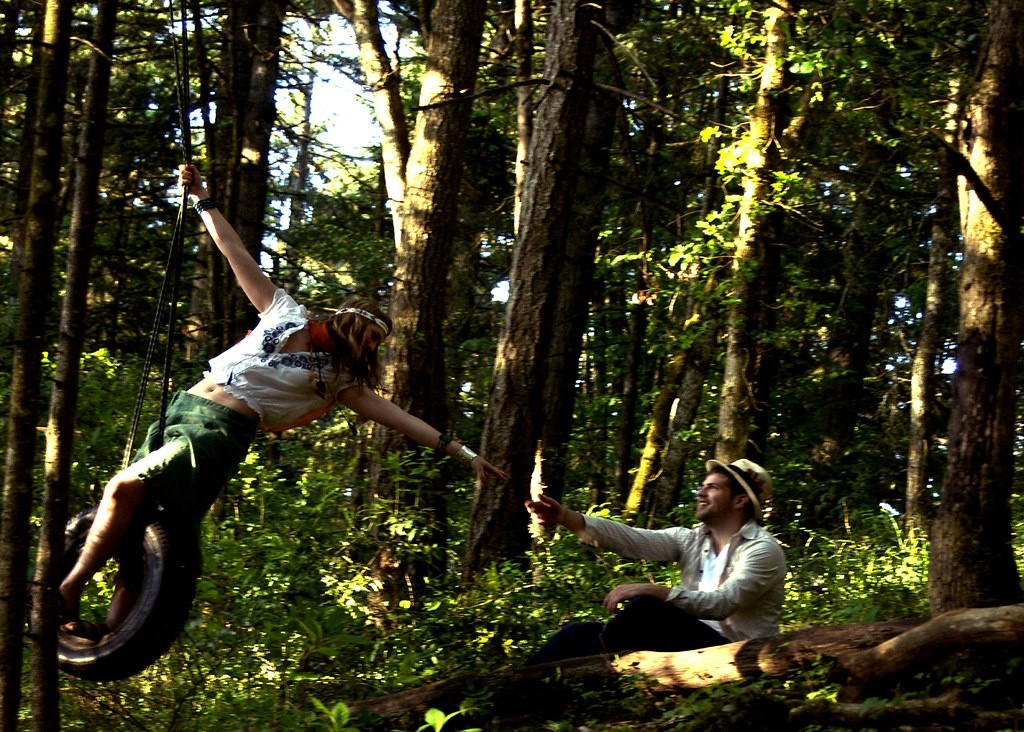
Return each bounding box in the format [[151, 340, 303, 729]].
[[523, 459, 786, 668], [59, 164, 508, 640]]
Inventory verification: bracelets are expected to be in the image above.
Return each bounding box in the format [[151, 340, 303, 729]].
[[195, 196, 218, 214], [561, 509, 568, 526], [453, 445, 478, 467], [435, 434, 454, 455]]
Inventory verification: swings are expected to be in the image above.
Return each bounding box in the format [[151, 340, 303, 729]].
[[53, 0, 209, 687]]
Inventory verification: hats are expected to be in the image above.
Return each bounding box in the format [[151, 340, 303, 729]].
[[706, 458, 771, 520]]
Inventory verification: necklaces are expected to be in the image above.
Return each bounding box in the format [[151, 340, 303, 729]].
[[307, 321, 358, 437]]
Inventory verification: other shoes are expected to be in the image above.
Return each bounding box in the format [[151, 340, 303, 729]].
[[43, 588, 79, 619], [60, 621, 109, 642]]
